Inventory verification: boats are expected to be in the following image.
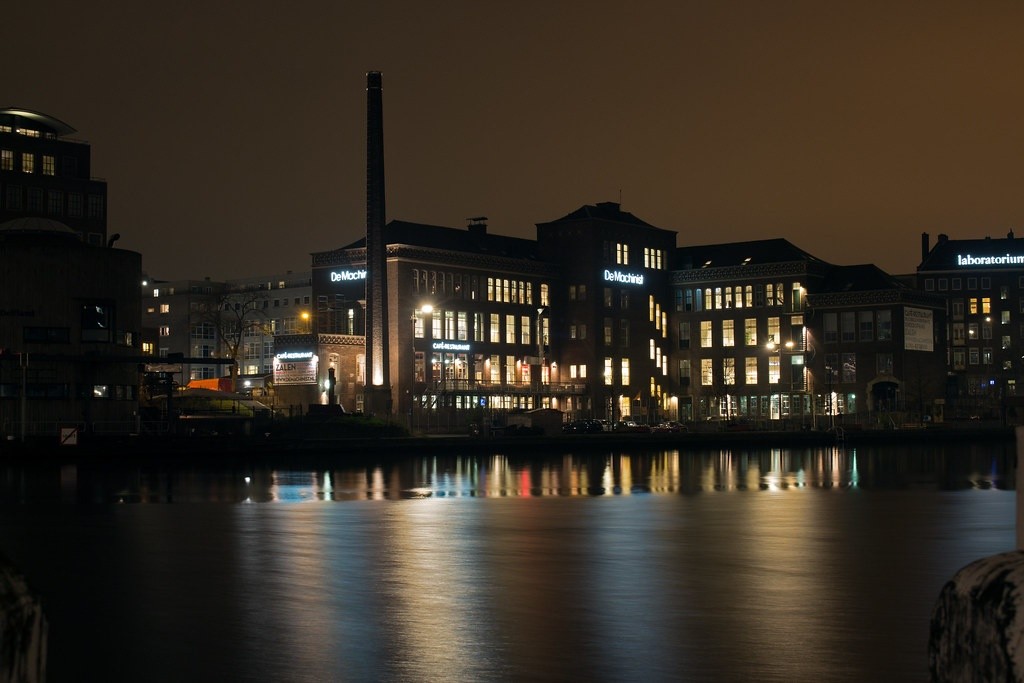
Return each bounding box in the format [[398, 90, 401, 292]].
[[120, 373, 1024, 489]]
